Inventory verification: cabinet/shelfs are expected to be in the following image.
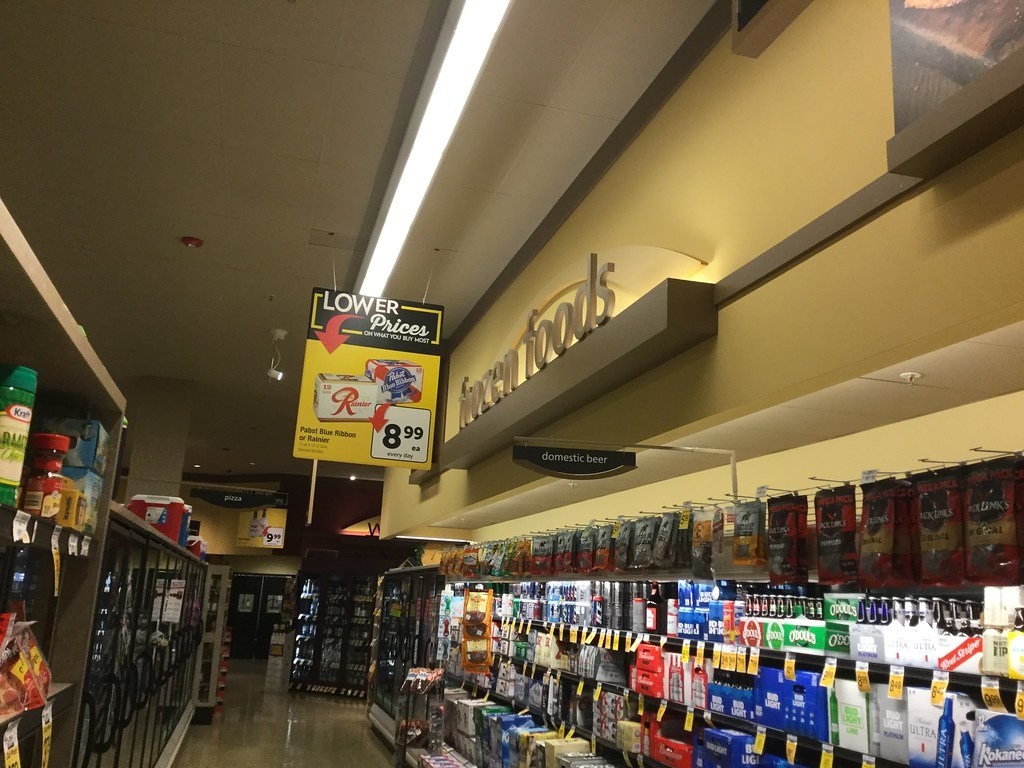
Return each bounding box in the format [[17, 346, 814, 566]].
[[0, 203, 129, 768], [405, 451, 1022, 768]]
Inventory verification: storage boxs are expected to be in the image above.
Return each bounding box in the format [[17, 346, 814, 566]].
[[443, 660, 697, 768], [0, 383, 112, 536]]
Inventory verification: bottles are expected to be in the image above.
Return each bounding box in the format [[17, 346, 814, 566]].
[[0, 364, 89, 535], [266, 633, 286, 679]]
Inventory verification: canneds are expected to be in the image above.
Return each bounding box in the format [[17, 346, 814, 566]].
[[0, 361, 69, 522]]
